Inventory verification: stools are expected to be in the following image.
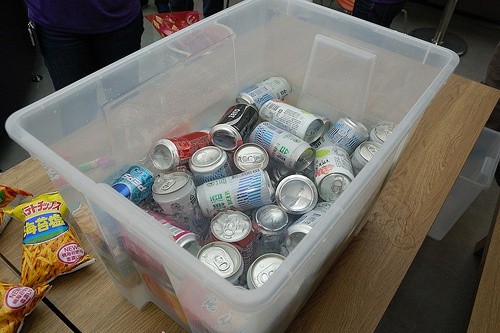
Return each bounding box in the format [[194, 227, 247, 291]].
[[408, 0, 468, 58]]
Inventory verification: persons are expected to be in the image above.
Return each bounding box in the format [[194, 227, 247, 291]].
[[22, 0, 145, 138]]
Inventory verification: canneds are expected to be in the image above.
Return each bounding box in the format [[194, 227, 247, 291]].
[[90, 76, 399, 333]]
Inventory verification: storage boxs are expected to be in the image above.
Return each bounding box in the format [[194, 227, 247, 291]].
[[0, 0, 460, 333], [428, 127, 500, 242]]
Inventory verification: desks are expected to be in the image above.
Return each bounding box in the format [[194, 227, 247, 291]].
[[0, 72, 500, 333], [467, 194, 500, 333]]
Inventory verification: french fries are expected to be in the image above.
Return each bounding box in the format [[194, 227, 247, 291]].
[[0, 282, 51, 333], [20, 231, 89, 285]]
